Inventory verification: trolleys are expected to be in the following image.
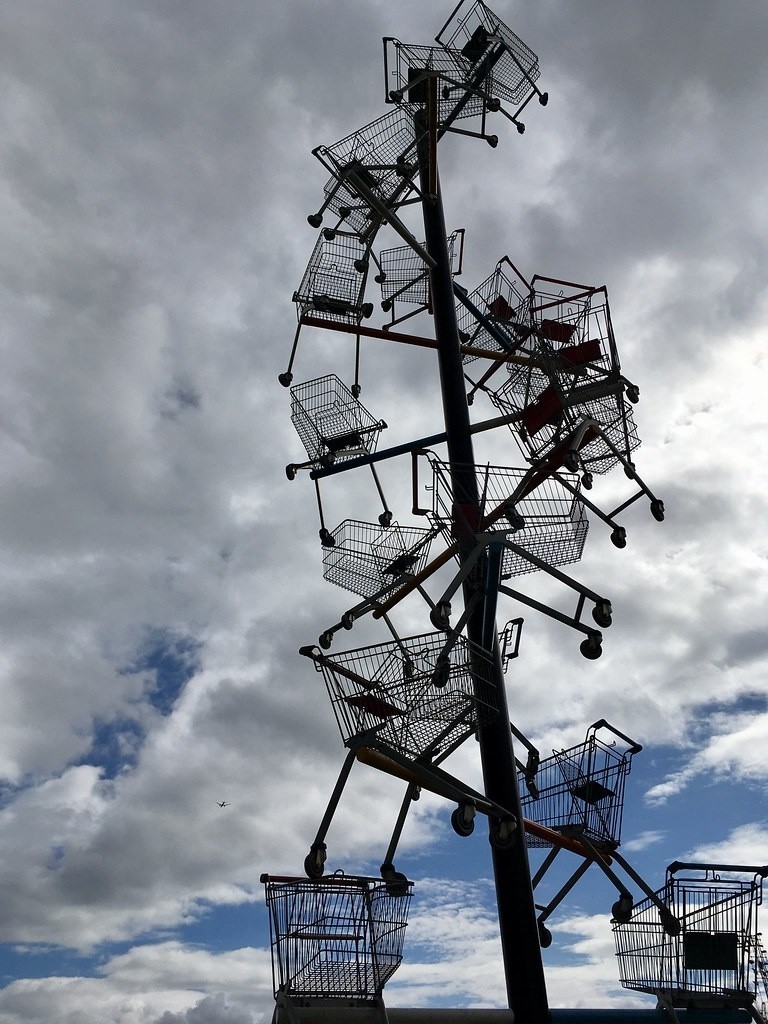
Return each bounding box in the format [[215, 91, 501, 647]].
[[261, 2, 768, 1024]]
